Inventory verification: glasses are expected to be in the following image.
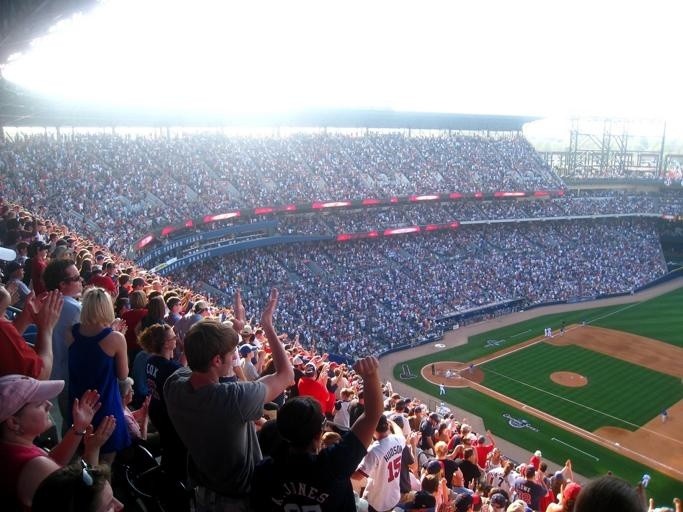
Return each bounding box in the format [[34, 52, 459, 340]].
[[66, 276, 79, 281], [79, 459, 93, 498]]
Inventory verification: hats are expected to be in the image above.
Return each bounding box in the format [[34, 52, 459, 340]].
[[239, 325, 258, 357], [430, 412, 439, 422], [0, 374, 65, 423], [330, 362, 339, 367], [293, 355, 317, 374], [455, 493, 480, 508], [193, 301, 208, 313], [564, 483, 581, 500]]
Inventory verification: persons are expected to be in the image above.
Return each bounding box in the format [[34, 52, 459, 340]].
[[1, 130, 682, 510]]
